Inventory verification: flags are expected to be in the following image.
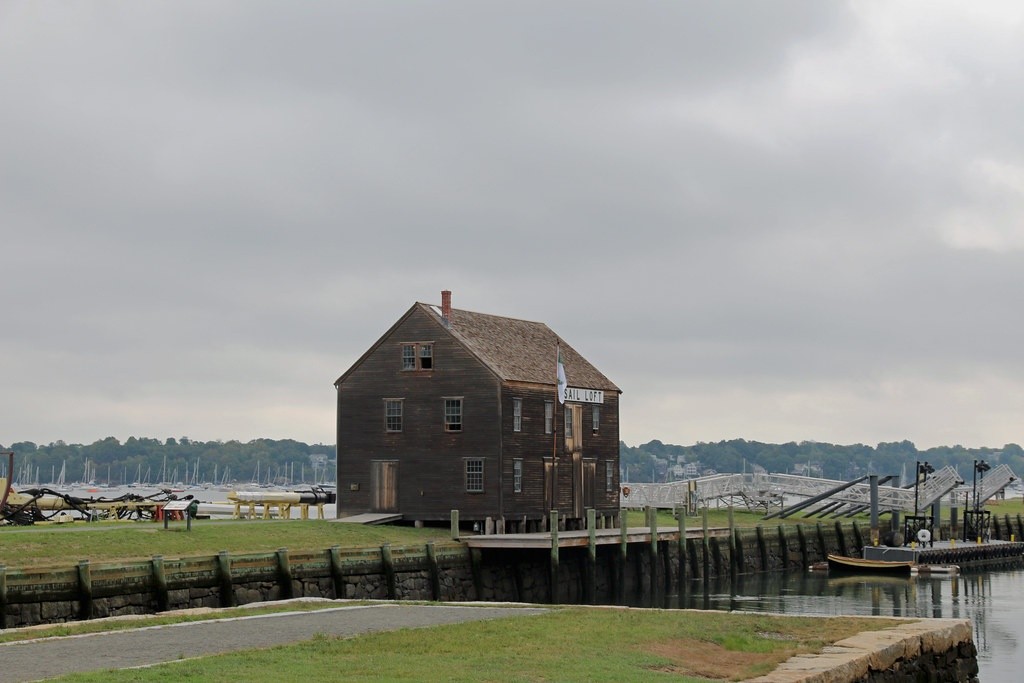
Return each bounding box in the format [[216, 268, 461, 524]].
[[557, 344, 568, 404]]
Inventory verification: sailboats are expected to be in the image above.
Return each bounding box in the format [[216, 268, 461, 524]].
[[1, 456, 337, 492]]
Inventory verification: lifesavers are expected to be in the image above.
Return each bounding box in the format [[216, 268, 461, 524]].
[[918, 529, 930, 542], [623, 487, 630, 494]]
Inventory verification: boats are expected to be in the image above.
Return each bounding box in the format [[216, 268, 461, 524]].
[[809, 562, 829, 570], [828, 459, 1024, 573]]
